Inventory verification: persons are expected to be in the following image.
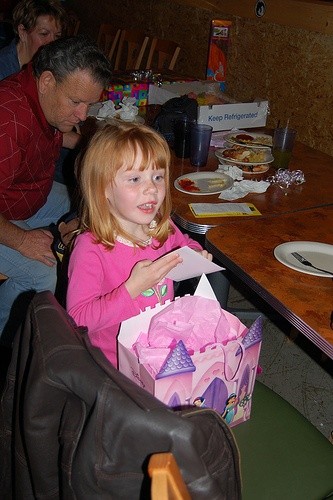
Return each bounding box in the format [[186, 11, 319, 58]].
[[1, 37, 111, 346], [66, 122, 212, 373], [0, 0, 67, 81]]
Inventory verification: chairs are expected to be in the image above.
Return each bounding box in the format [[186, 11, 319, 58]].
[[97, 22, 181, 72], [28, 292, 333, 500]]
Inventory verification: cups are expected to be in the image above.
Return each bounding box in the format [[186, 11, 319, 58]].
[[173, 115, 196, 159], [271, 128, 297, 169], [189, 124, 213, 167]]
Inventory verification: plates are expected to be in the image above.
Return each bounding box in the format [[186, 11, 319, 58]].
[[274, 241, 333, 278], [174, 172, 234, 195], [218, 158, 270, 174], [214, 149, 274, 165], [224, 133, 273, 148]]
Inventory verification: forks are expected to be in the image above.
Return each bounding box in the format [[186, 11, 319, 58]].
[[232, 137, 273, 148], [291, 252, 333, 275]]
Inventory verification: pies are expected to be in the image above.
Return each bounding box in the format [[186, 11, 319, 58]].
[[235, 134, 254, 142]]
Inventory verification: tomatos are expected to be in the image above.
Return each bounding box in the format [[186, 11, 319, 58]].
[[244, 158, 249, 163]]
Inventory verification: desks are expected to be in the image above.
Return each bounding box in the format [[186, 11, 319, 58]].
[[111, 69, 333, 359]]
